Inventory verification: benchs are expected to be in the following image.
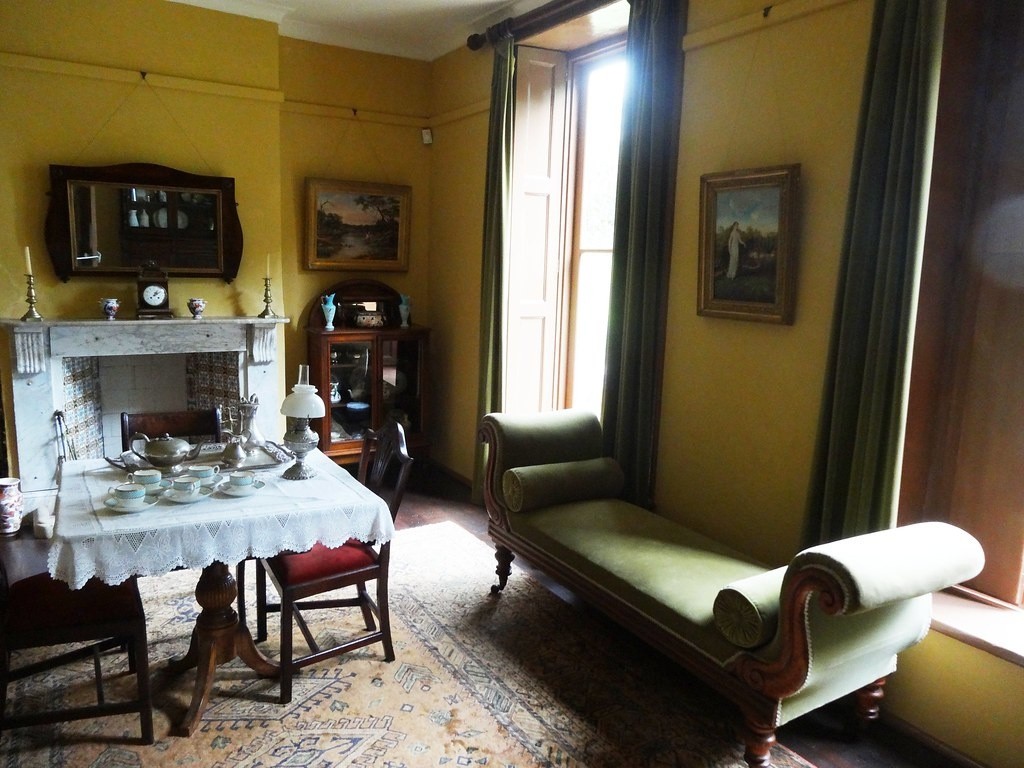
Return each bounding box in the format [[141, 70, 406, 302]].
[[476, 409, 986, 768]]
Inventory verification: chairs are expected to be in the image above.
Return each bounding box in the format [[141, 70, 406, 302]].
[[254, 420, 414, 704], [120, 408, 221, 452], [0, 569, 157, 748]]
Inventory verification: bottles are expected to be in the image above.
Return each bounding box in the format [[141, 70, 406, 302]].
[[0, 477, 22, 536], [127, 187, 191, 228]]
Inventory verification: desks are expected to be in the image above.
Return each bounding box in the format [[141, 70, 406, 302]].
[[46, 437, 396, 741]]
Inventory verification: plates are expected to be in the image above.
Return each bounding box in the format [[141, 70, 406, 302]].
[[104, 493, 160, 512], [163, 485, 213, 503], [153, 210, 161, 228], [385, 370, 407, 394], [180, 473, 224, 486], [158, 207, 189, 229], [218, 480, 267, 497], [116, 478, 173, 492]]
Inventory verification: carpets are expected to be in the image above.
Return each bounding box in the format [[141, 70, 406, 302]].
[[0, 516, 821, 768]]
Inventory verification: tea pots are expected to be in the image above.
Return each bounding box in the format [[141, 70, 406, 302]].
[[128, 432, 205, 472], [330, 381, 342, 403], [220, 394, 259, 467], [348, 376, 368, 402]]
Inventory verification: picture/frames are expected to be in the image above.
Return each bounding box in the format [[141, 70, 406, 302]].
[[302, 177, 414, 275], [697, 162, 803, 327]]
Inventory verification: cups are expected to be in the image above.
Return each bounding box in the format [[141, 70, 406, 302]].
[[108, 483, 146, 507], [172, 476, 201, 498], [189, 465, 220, 484], [229, 471, 255, 491], [126, 469, 162, 490]]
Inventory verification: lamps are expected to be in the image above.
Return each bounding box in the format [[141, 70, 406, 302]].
[[280, 363, 325, 482]]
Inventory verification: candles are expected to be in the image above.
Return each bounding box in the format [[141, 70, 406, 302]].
[[266, 253, 270, 277], [24, 246, 33, 275]]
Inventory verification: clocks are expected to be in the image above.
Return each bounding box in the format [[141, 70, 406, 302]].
[[136, 259, 176, 320]]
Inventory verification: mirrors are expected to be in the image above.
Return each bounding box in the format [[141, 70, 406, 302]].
[[43, 162, 244, 286]]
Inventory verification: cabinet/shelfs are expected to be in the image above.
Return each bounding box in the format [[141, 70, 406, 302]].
[[301, 277, 433, 466], [120, 187, 218, 269]]
